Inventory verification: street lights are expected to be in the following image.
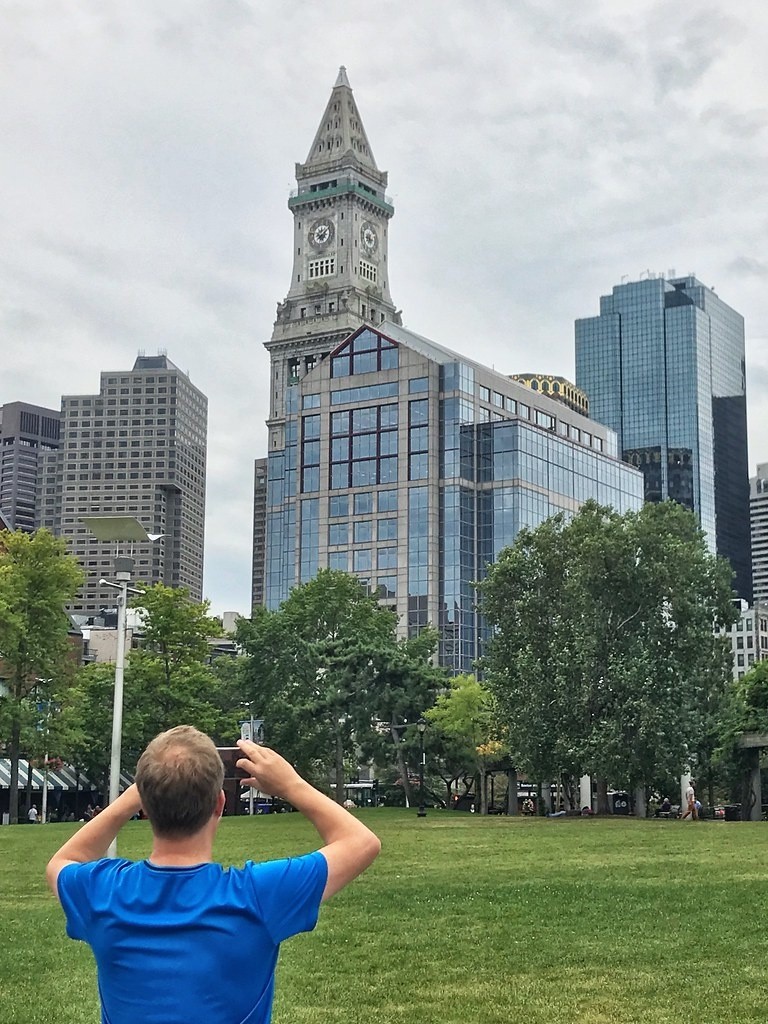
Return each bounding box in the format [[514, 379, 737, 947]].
[[416, 718, 427, 817], [78, 515, 167, 860]]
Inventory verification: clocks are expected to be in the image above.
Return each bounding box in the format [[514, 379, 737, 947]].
[[361, 221, 379, 254], [308, 218, 335, 251]]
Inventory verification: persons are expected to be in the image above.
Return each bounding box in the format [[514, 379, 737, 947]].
[[45, 724, 383, 1024], [27, 804, 103, 824], [655, 798, 671, 818], [522, 798, 534, 816], [695, 798, 703, 820], [680, 781, 696, 820]]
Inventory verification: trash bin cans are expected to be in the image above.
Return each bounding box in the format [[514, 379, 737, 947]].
[[613, 793, 630, 815], [724, 806, 738, 822]]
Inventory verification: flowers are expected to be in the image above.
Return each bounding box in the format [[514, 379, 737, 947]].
[[45, 756, 63, 772], [29, 758, 44, 769]]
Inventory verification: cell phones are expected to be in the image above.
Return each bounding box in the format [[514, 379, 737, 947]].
[[216, 746, 251, 780]]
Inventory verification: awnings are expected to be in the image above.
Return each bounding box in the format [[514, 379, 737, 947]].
[[0, 759, 136, 791]]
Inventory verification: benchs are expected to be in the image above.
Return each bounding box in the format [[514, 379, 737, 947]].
[[488, 809, 536, 817], [659, 805, 682, 819]]
